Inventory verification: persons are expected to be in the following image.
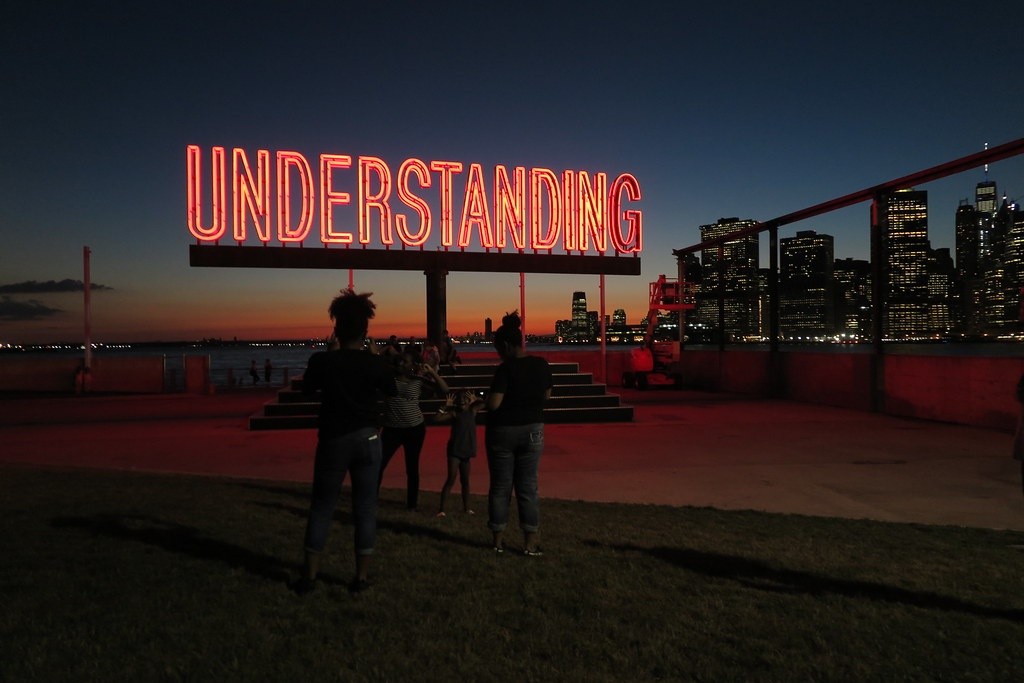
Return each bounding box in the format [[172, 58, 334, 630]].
[[483, 314, 552, 558], [434, 389, 485, 517], [294, 289, 386, 594], [249, 357, 271, 385], [630, 339, 653, 393], [367, 340, 449, 512], [386, 330, 463, 373]]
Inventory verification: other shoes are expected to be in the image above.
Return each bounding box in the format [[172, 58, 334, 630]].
[[436, 511, 448, 519], [353, 578, 370, 592], [305, 578, 317, 590], [466, 509, 475, 515]]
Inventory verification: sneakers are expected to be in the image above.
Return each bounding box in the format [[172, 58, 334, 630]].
[[493, 546, 504, 553], [524, 547, 544, 556]]
